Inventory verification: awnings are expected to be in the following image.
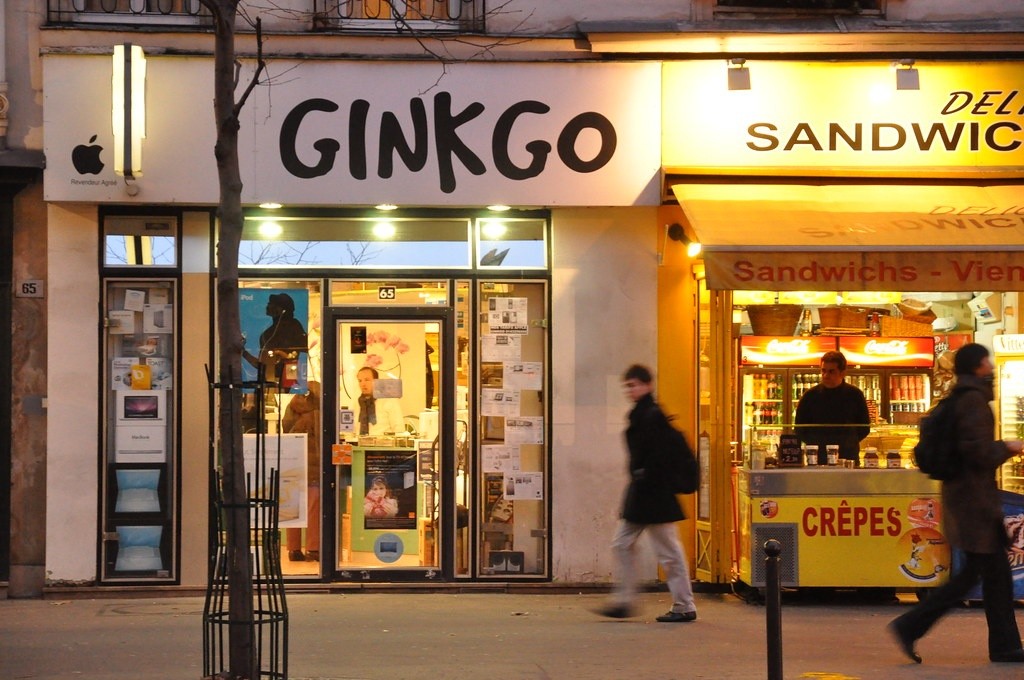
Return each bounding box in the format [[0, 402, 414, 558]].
[[670, 182, 1024, 292]]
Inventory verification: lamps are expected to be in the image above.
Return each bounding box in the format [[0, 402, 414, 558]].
[[112, 42, 147, 196], [727, 59, 750, 91], [668, 223, 701, 257], [897, 61, 920, 91]]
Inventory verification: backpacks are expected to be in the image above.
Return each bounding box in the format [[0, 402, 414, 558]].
[[640, 407, 700, 494], [913, 388, 976, 481]]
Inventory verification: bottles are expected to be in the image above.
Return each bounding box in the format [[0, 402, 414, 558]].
[[742, 369, 783, 461], [751, 446, 766, 470], [886, 450, 901, 469], [863, 448, 880, 468]]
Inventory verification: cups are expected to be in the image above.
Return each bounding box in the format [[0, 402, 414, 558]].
[[806, 445, 819, 465], [825, 444, 840, 466]]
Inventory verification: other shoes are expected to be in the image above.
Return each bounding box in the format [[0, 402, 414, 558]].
[[289, 550, 304, 561], [596, 602, 636, 618], [304, 550, 319, 562], [887, 620, 922, 665], [655, 610, 696, 623]]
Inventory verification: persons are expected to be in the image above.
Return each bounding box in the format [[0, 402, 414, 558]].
[[349, 366, 408, 438], [282, 380, 321, 563], [588, 364, 705, 621], [363, 475, 400, 519], [794, 351, 870, 467], [885, 343, 1024, 663]]
